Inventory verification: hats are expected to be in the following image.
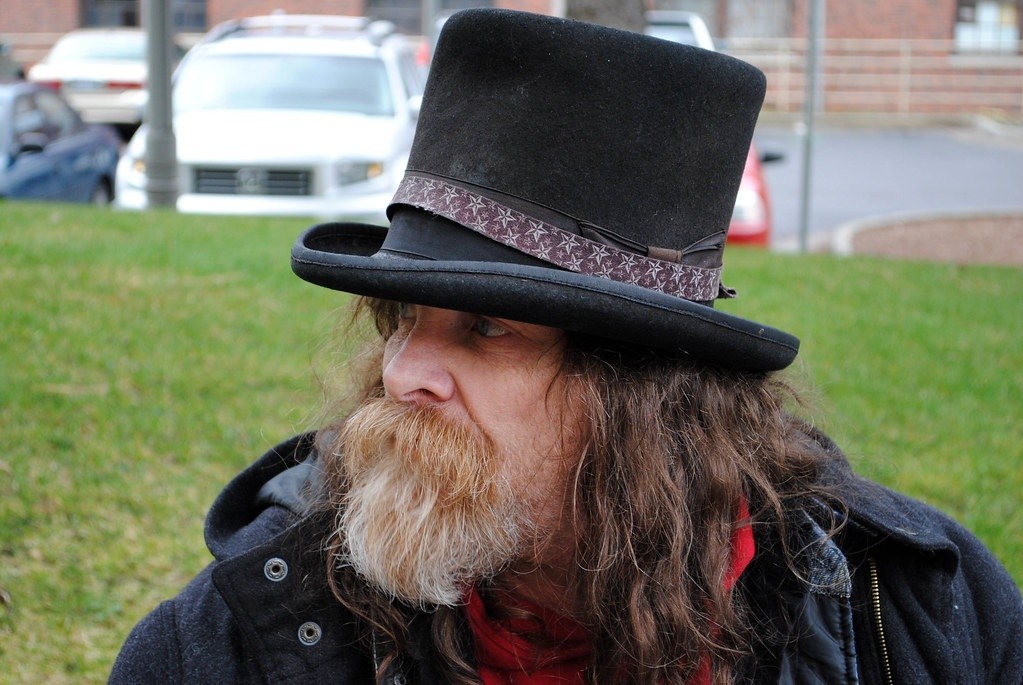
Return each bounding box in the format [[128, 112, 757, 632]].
[[289, 6, 801, 372]]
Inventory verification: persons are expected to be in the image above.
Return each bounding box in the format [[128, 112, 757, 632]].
[[109, 7, 1023, 685]]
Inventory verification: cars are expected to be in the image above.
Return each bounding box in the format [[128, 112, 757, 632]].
[[640, 11, 787, 248], [26, 27, 185, 140]]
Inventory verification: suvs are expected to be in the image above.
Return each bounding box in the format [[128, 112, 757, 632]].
[[114, 13, 431, 224]]
